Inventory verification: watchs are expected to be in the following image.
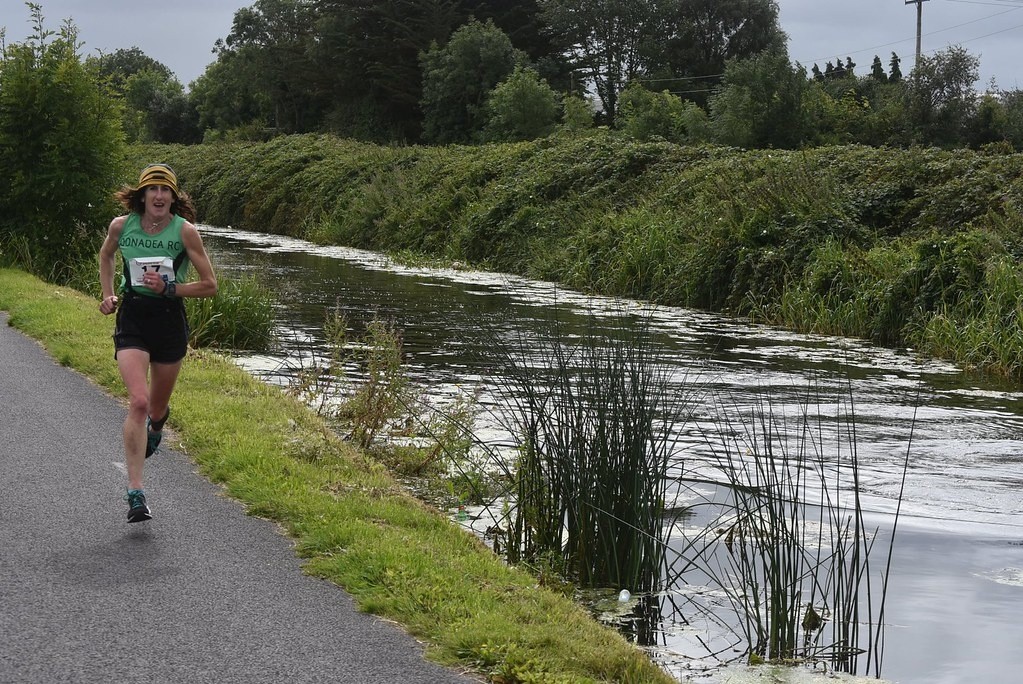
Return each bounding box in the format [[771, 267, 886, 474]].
[[163, 280, 176, 300]]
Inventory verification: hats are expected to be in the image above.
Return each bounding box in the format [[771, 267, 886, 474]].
[[137, 163, 178, 197]]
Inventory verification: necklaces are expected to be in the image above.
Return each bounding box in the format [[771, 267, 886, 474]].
[[142, 213, 170, 231]]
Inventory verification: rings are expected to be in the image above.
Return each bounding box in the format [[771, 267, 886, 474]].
[[148, 278, 150, 284]]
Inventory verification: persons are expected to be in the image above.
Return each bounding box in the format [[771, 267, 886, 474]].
[[99, 163, 218, 523]]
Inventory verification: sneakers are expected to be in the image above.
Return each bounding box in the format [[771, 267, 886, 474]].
[[123, 487, 152, 523], [145, 405, 170, 458]]
